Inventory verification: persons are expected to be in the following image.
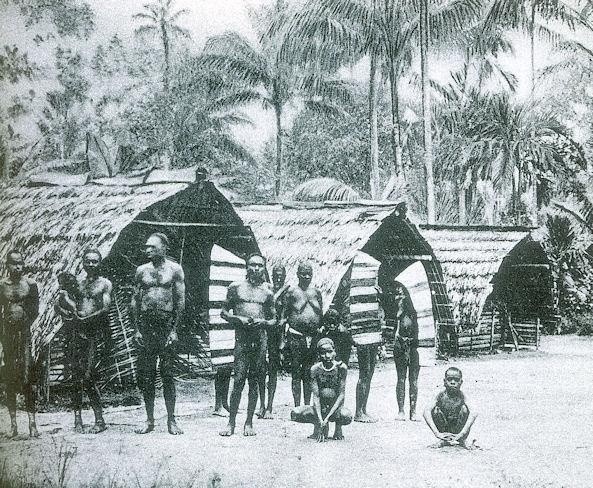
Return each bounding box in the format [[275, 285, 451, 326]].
[[53, 248, 113, 434], [0, 249, 40, 439], [423, 367, 478, 451], [130, 233, 185, 435], [213, 254, 420, 444]]
